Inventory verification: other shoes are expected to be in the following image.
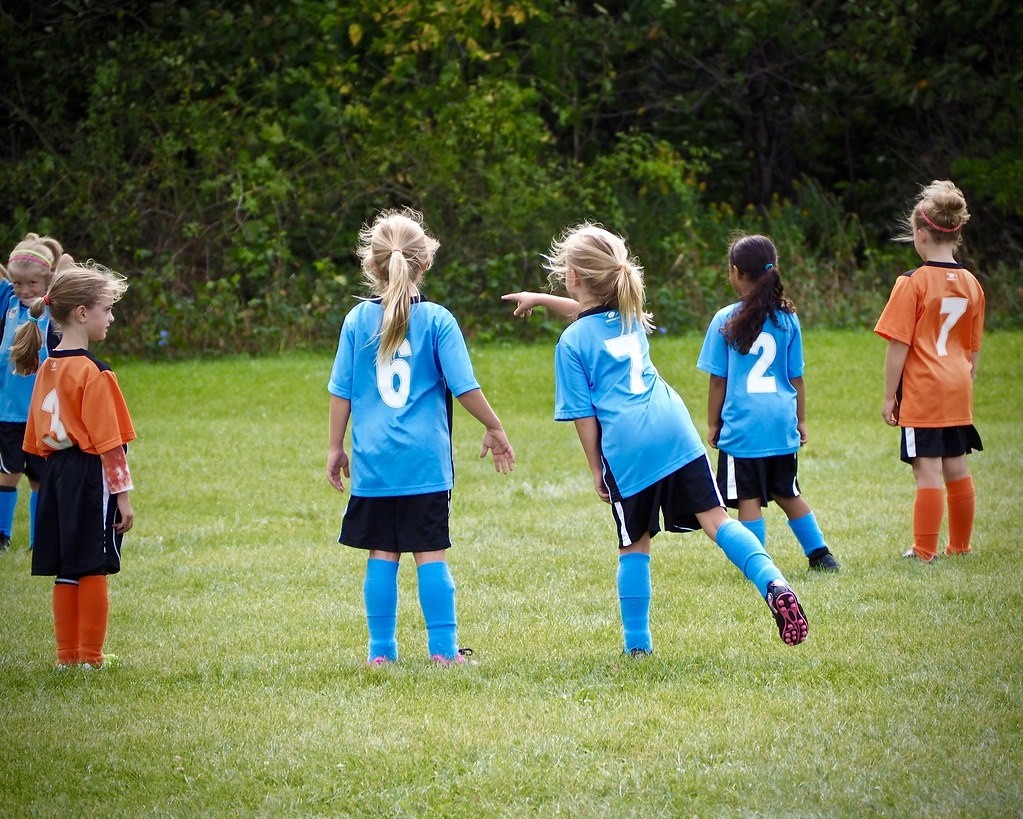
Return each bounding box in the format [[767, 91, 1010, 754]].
[[0, 539, 11, 556], [56, 655, 120, 672]]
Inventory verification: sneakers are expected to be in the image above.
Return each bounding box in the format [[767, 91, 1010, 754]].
[[806, 553, 840, 573], [366, 655, 391, 667], [431, 650, 477, 666], [765, 579, 809, 647], [619, 647, 653, 659], [901, 549, 941, 563]]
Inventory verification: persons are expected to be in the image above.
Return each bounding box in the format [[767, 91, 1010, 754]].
[[500, 219, 808, 665], [7, 257, 137, 673], [873, 178, 984, 564], [326, 206, 515, 669], [0, 233, 78, 551], [696, 233, 841, 577]]
